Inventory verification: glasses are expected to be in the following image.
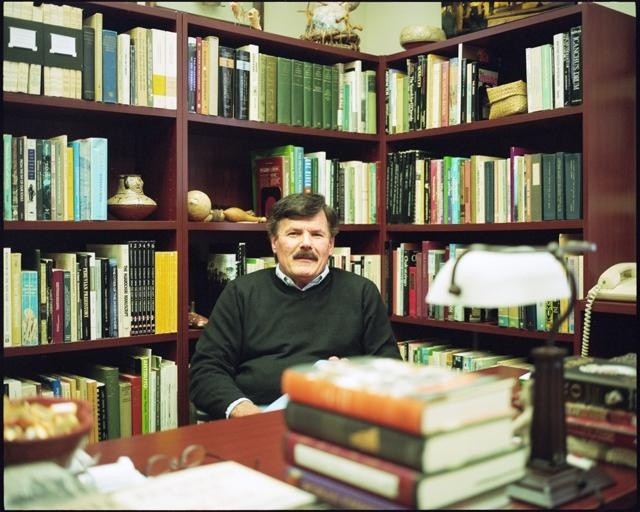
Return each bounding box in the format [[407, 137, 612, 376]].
[[144, 444, 260, 475]]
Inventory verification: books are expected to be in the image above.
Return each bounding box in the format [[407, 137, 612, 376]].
[[384, 234, 584, 333], [386, 150, 583, 225], [251, 145, 377, 224], [3, 134, 107, 220], [281, 358, 530, 511], [188, 36, 377, 134], [191, 241, 381, 318], [398, 332, 637, 468], [4, 346, 179, 444], [385, 25, 582, 134], [3, 2, 177, 110], [5, 240, 178, 348]]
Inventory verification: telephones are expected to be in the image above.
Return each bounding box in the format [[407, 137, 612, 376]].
[[588, 261, 637, 302]]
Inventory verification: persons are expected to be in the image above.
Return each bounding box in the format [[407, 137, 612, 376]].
[[190, 197, 402, 423]]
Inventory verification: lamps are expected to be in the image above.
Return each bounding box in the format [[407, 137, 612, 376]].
[[424, 241, 618, 507]]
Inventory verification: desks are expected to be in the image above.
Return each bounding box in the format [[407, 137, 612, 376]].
[[3, 410, 636, 512]]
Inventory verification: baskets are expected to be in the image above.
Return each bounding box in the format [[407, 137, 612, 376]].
[[486, 80, 528, 120]]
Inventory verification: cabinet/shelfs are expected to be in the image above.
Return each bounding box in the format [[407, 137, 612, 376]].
[[1, 1, 183, 442], [181, 9, 385, 427], [380, 2, 638, 378]]
